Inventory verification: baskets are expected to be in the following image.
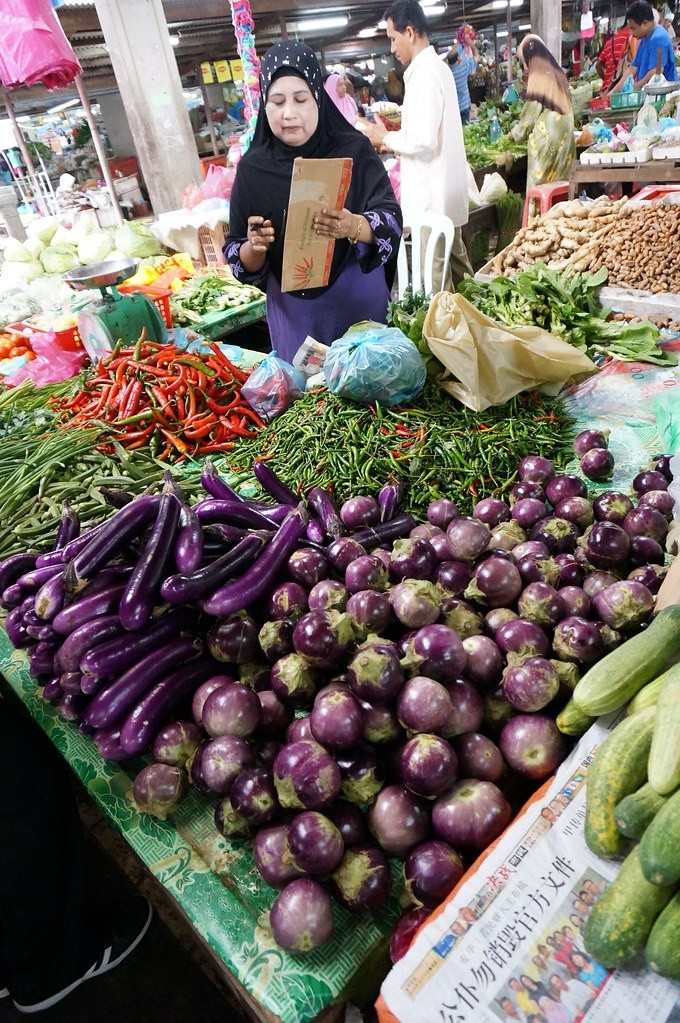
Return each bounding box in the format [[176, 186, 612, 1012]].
[[4, 284, 173, 354], [199, 220, 231, 268], [175, 243, 207, 269], [610, 90, 643, 108], [589, 95, 609, 110]]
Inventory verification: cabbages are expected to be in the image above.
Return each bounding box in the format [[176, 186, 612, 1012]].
[[0, 215, 167, 297]]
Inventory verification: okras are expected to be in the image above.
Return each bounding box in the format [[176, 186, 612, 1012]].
[[0, 448, 218, 563]]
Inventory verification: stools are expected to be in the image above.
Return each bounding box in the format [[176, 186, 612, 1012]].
[[522, 181, 569, 228]]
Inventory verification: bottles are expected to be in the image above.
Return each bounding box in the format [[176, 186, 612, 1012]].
[[490, 115, 503, 144]]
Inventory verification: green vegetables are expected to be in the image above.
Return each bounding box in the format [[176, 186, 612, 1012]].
[[381, 262, 679, 369], [165, 274, 263, 327], [459, 97, 529, 256]]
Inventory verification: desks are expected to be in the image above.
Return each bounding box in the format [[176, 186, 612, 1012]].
[[569, 158, 680, 202]]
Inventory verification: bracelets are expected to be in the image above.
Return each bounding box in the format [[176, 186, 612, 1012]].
[[348, 216, 362, 245], [508, 132, 511, 134]]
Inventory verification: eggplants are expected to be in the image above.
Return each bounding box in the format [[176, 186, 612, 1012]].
[[268, 877, 335, 953], [0, 428, 674, 910], [388, 901, 434, 964]]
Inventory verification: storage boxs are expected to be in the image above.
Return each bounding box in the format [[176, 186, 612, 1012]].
[[119, 188, 144, 216], [95, 155, 141, 183], [97, 174, 144, 195], [200, 154, 226, 180]]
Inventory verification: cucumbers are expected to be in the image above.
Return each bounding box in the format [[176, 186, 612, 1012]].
[[556, 603, 680, 979]]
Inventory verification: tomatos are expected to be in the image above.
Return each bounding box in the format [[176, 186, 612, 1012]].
[[0, 332, 38, 362]]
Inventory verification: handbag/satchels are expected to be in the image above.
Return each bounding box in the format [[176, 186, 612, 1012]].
[[323, 327, 426, 405], [575, 118, 680, 152], [240, 349, 306, 419], [422, 291, 596, 413]]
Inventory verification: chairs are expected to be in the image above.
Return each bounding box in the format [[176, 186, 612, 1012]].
[[395, 213, 455, 303]]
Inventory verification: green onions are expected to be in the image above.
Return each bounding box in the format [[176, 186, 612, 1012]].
[[0, 419, 129, 515]]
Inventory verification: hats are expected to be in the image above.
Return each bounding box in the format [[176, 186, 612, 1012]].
[[665, 14, 674, 20]]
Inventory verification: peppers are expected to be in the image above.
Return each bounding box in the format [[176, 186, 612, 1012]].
[[51, 326, 585, 523]]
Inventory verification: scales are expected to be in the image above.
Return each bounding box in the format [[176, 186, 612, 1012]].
[[499, 79, 520, 103], [636, 80, 680, 126], [61, 257, 170, 364]]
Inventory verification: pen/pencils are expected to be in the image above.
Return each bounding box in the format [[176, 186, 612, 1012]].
[[250, 211, 272, 232]]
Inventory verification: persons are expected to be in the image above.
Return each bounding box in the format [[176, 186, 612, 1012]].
[[596, 1, 680, 97], [443, 33, 479, 125], [355, 0, 474, 292], [323, 59, 388, 126], [222, 39, 403, 364], [508, 34, 574, 224]]
